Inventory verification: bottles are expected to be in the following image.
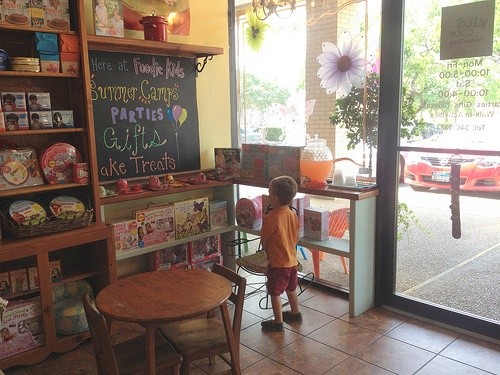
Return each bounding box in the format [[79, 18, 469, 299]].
[[302, 135, 333, 188]]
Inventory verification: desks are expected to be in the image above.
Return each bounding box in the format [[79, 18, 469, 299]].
[[95, 270, 241, 375]]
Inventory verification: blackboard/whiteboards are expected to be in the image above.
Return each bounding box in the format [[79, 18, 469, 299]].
[[89, 49, 201, 186]]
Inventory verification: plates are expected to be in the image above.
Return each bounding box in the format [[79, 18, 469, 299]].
[[49, 22, 70, 29], [7, 18, 30, 24]]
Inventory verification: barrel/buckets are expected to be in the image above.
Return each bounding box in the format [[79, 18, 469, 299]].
[[139, 15, 168, 42]]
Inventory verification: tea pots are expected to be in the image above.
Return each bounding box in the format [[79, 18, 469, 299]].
[[116, 172, 206, 193]]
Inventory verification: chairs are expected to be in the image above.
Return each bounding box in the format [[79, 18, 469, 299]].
[[225, 202, 315, 310], [161, 263, 247, 375], [82, 293, 183, 375], [310, 207, 350, 274]]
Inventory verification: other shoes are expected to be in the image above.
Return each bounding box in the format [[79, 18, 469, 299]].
[[261, 320, 283, 330], [282, 310, 302, 320]]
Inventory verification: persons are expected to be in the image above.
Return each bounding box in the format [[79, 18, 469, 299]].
[[0, 281, 10, 290], [54, 112, 66, 128], [95, 0, 107, 28], [6, 113, 18, 123], [261, 176, 302, 329], [3, 94, 16, 104], [145, 223, 154, 234], [31, 113, 43, 129], [111, 9, 123, 34], [1, 328, 14, 342], [29, 94, 42, 111], [138, 227, 145, 241], [51, 268, 61, 283], [215, 148, 225, 169]]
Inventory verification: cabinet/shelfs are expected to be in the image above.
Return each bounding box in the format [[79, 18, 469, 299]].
[[0, 0, 380, 375]]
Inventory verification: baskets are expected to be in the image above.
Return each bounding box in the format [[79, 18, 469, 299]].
[[0, 194, 95, 239]]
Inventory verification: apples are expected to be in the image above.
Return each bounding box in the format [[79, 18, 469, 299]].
[[164, 175, 174, 180]]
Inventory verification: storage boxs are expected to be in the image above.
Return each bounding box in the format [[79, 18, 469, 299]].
[[147, 242, 188, 271], [303, 206, 330, 241], [131, 203, 175, 248], [209, 200, 228, 232], [1, 92, 26, 111], [168, 196, 209, 240], [107, 217, 138, 255], [4, 111, 29, 131], [52, 110, 74, 129], [48, 258, 62, 284], [188, 233, 222, 264], [27, 266, 40, 291], [27, 93, 51, 111], [29, 110, 54, 129], [8, 268, 29, 293]]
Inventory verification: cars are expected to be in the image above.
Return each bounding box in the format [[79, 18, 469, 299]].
[[402, 119, 500, 193]]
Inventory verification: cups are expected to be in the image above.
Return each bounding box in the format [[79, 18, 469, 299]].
[[333, 168, 358, 187]]
